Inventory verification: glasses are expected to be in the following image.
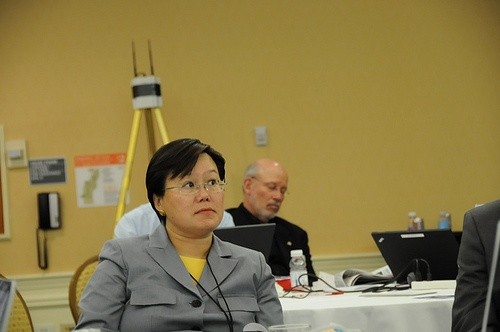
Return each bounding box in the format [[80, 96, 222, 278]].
[[252, 176, 291, 199], [163, 181, 227, 195]]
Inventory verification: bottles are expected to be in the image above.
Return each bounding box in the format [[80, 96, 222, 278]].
[[289, 249, 309, 287], [408, 211, 424, 231], [439, 211, 451, 231]]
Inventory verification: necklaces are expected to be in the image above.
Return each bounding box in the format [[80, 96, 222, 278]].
[[189, 251, 234, 332]]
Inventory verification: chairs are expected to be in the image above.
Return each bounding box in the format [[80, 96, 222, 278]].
[[69, 255, 100, 325], [0, 273, 34, 332]]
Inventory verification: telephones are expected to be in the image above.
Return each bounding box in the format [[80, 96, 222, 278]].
[[38, 192, 60, 230]]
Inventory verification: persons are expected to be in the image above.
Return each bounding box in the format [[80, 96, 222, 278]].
[[112, 203, 235, 239], [72, 138, 287, 332], [451, 199, 500, 332], [224, 159, 318, 287]]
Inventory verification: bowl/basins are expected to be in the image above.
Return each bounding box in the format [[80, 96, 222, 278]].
[[275, 278, 291, 291]]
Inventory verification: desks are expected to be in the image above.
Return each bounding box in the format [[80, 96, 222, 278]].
[[278, 279, 457, 332]]
